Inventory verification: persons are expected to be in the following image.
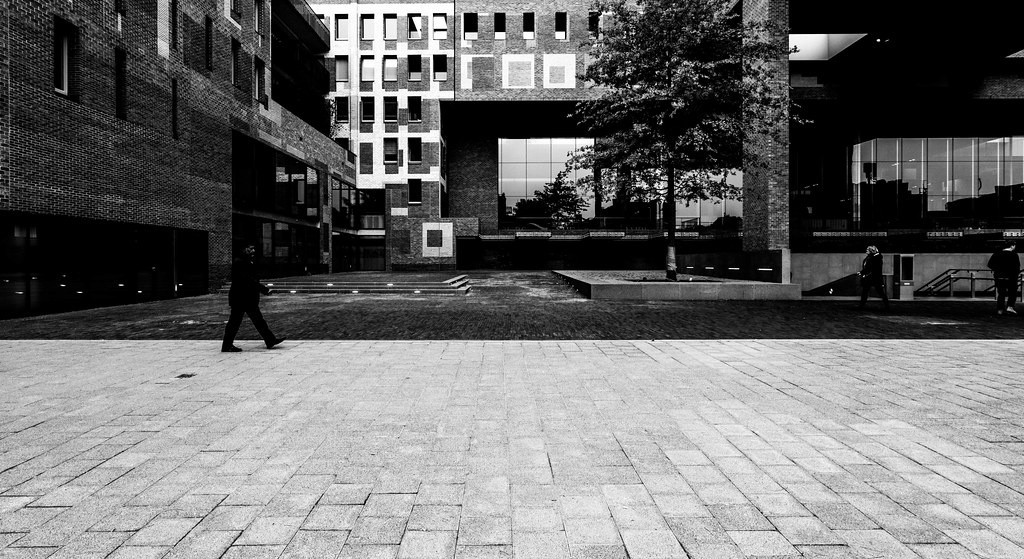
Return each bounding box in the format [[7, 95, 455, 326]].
[[988, 240, 1020, 315], [220, 243, 286, 352], [857, 246, 888, 302]]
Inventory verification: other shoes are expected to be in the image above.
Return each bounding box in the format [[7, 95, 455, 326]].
[[221, 343, 242, 352], [998, 310, 1003, 314], [268, 336, 286, 348], [1006, 306, 1016, 313]]
